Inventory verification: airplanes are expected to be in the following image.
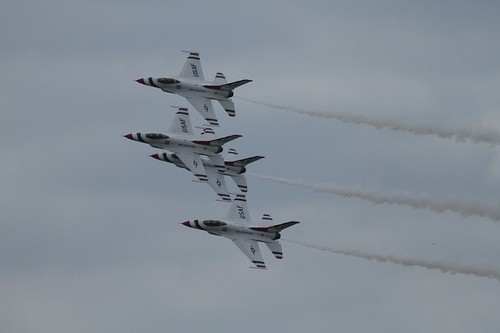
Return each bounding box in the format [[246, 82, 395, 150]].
[[124, 108, 243, 182], [135, 50, 251, 126], [181, 195, 300, 269], [148, 152, 265, 201]]
